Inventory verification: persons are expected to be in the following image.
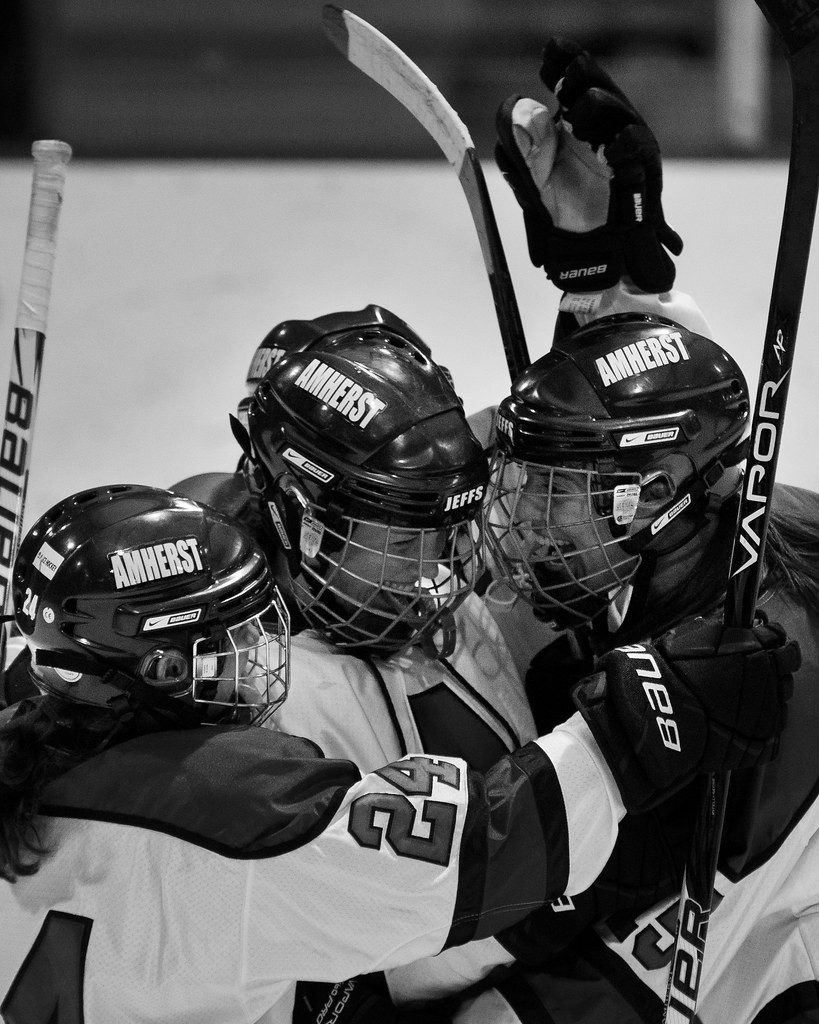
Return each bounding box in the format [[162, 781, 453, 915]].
[[174, 36, 712, 1023], [495, 317, 819, 1024], [0, 483, 802, 1024]]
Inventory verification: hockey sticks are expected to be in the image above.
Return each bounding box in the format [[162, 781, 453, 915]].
[[656, 1, 819, 1024], [0, 139, 75, 677], [319, 1, 534, 383]]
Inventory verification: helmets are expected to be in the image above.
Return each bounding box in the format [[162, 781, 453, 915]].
[[232, 303, 490, 560], [12, 485, 276, 706], [499, 308, 752, 484]]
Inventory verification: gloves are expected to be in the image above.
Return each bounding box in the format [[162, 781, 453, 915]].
[[573, 608, 802, 814], [493, 41, 682, 297]]
